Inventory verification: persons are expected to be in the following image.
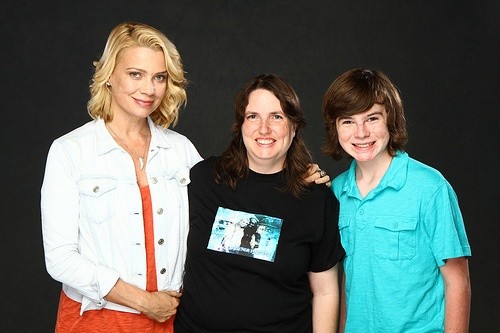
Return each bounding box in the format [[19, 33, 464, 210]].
[[41, 21, 331, 333], [174, 75, 347, 333], [237, 218, 260, 252], [322, 68, 471, 333]]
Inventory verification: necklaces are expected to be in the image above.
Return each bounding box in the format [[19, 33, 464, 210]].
[[108, 124, 149, 171]]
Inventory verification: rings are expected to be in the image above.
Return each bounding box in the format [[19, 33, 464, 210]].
[[318, 170, 327, 178]]
